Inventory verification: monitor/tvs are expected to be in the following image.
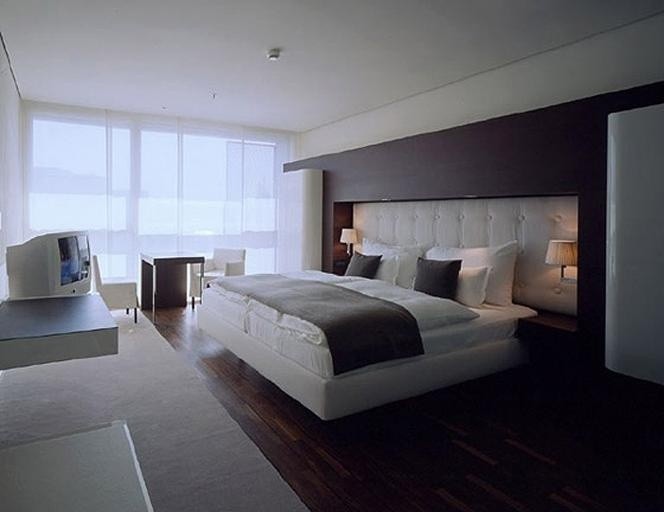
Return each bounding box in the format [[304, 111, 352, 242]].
[[5, 230, 92, 301]]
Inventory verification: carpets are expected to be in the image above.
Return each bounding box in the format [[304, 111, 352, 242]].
[[1, 305, 312, 511]]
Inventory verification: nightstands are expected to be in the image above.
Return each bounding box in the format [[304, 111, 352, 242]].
[[514, 316, 575, 356]]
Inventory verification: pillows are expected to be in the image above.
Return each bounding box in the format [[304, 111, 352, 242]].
[[345, 235, 519, 307]]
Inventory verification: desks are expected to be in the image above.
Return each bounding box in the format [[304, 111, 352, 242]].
[[0, 292, 119, 370], [139, 250, 205, 323]]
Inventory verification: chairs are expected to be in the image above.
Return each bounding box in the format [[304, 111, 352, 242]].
[[92, 254, 140, 324], [190, 247, 246, 309]]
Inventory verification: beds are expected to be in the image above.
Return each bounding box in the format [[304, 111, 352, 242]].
[[197, 195, 579, 422]]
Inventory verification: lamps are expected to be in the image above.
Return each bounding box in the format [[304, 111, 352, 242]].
[[544, 239, 577, 279], [340, 228, 356, 256]]
